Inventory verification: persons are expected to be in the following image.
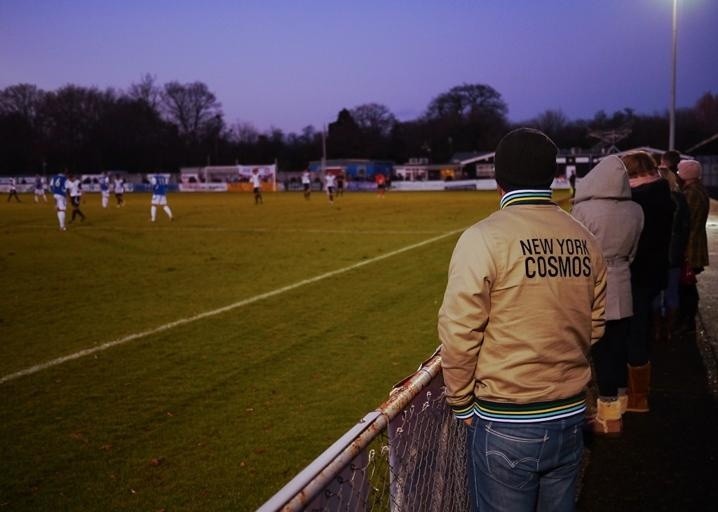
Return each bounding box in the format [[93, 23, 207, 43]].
[[569, 148, 710, 436], [436, 129, 608, 511], [6, 167, 182, 231], [189, 169, 425, 204], [444, 171, 471, 181]]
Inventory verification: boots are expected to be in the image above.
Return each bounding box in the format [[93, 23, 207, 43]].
[[584, 360, 650, 438]]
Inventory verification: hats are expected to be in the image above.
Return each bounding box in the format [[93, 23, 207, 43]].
[[678, 160, 703, 178], [495, 128, 557, 188]]
